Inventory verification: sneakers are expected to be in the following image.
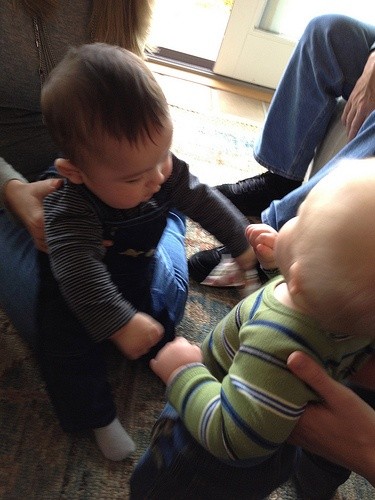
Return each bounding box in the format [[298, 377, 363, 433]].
[[214, 170, 302, 217], [188, 244, 268, 288]]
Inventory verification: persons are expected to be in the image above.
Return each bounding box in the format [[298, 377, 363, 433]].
[[281, 342, 375, 500], [0, 0, 189, 384], [127, 157, 375, 500], [39, 41, 260, 463], [185, 12, 375, 288]]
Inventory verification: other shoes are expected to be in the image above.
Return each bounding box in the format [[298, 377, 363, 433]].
[[287, 452, 349, 500]]
